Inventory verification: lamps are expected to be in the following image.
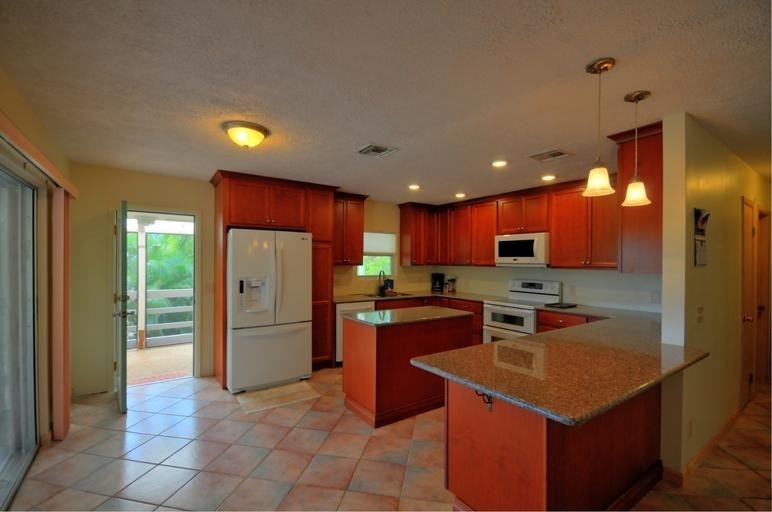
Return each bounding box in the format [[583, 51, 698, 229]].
[[221, 120, 269, 147], [621, 90, 652, 207], [581, 57, 616, 197]]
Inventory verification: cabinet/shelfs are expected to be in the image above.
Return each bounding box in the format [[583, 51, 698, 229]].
[[435, 296, 449, 309], [304, 181, 341, 242], [397, 201, 432, 265], [497, 186, 549, 234], [432, 203, 450, 266], [448, 297, 482, 345], [549, 173, 618, 268], [422, 295, 435, 306], [312, 242, 336, 368], [209, 168, 306, 231], [375, 296, 424, 311], [334, 191, 370, 265], [451, 194, 497, 266], [537, 310, 588, 333], [606, 119, 662, 274]]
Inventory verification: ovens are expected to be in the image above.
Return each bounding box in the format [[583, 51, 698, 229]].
[[482, 300, 537, 346]]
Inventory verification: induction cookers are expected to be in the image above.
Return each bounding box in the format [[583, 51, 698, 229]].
[[479, 297, 557, 307]]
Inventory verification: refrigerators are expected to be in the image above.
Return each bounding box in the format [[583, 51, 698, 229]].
[[223, 226, 315, 395]]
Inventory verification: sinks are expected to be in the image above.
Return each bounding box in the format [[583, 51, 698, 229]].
[[366, 290, 412, 298]]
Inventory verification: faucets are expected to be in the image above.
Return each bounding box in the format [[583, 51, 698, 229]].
[[379, 311, 388, 320], [378, 269, 388, 293]]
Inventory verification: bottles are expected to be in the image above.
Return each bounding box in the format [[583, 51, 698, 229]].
[[443, 278, 456, 297]]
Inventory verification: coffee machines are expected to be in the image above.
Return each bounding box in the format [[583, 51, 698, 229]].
[[431, 272, 445, 294]]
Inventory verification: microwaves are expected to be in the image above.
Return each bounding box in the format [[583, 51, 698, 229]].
[[494, 233, 550, 269]]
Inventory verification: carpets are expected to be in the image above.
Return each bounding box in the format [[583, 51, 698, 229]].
[[237, 378, 320, 415]]
